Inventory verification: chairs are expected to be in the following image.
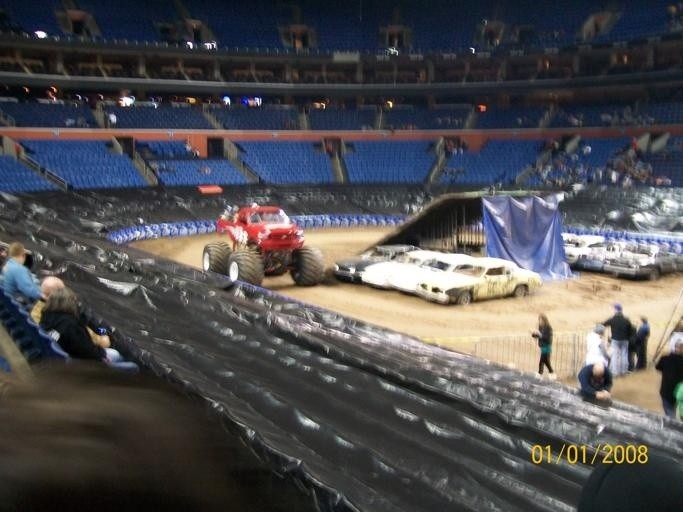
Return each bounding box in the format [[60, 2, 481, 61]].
[[1, 264, 76, 378]]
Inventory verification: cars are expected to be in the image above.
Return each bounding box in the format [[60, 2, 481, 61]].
[[332, 243, 543, 305], [560, 232, 678, 280]]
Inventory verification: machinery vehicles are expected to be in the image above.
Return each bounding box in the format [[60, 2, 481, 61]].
[[203, 205, 325, 287]]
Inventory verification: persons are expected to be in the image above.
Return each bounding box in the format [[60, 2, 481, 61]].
[[656, 332, 683, 419], [579, 363, 613, 400], [578, 454, 682, 512], [532, 314, 555, 378], [0, 362, 275, 510], [0, 239, 139, 370], [602, 304, 631, 376], [635, 315, 650, 369], [673, 383, 683, 421], [587, 324, 611, 368], [669, 319, 683, 354], [0, 1, 683, 189], [626, 319, 637, 371]]
